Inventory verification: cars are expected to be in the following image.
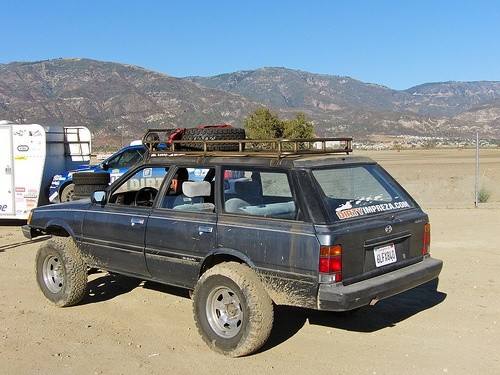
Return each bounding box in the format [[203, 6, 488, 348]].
[[49, 140, 249, 204]]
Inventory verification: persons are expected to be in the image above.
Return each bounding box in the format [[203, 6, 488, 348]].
[[153, 166, 201, 208]]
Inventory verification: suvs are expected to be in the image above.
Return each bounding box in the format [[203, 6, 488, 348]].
[[20, 129, 444, 358]]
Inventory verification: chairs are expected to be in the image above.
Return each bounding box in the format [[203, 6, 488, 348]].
[[172, 182, 216, 212], [241, 201, 295, 216], [226, 180, 263, 206]]
